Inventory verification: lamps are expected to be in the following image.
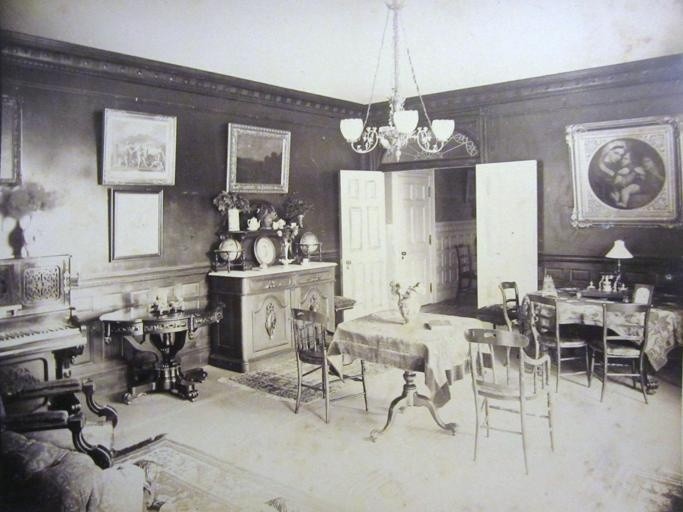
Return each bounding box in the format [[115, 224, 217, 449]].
[[605, 239, 634, 281], [340, 0, 455, 164]]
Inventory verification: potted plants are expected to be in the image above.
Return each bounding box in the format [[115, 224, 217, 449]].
[[213, 191, 315, 232], [388, 279, 427, 324], [0, 182, 72, 258]]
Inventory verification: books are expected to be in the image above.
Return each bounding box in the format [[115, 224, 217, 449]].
[[430, 320, 452, 331]]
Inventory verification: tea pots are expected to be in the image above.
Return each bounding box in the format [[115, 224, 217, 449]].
[[247, 217, 261, 231]]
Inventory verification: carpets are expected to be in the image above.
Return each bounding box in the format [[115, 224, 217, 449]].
[[217, 357, 399, 406], [111, 432, 349, 512]]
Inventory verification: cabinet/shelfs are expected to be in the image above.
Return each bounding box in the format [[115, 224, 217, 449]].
[[207, 229, 338, 373]]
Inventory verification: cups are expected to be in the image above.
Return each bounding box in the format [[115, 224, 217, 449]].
[[297, 214, 304, 229]]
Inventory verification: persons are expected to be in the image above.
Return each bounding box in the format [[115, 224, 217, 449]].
[[115, 137, 165, 172], [595, 141, 664, 208]]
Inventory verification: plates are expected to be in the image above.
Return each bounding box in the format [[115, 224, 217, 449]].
[[254, 236, 277, 265]]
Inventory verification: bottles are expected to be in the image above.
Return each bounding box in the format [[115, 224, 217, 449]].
[[598, 274, 611, 291]]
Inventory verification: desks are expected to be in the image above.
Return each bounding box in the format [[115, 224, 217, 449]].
[[99, 298, 225, 405], [337, 309, 491, 443]]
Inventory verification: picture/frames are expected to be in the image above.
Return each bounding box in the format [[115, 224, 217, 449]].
[[565, 112, 683, 231], [109, 186, 164, 263], [225, 123, 292, 194], [101, 107, 178, 186], [0, 93, 24, 188]]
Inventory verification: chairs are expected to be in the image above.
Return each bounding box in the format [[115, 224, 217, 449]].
[[453, 244, 477, 302], [291, 307, 369, 424], [463, 328, 555, 475], [0, 376, 119, 469], [499, 271, 683, 404]]
